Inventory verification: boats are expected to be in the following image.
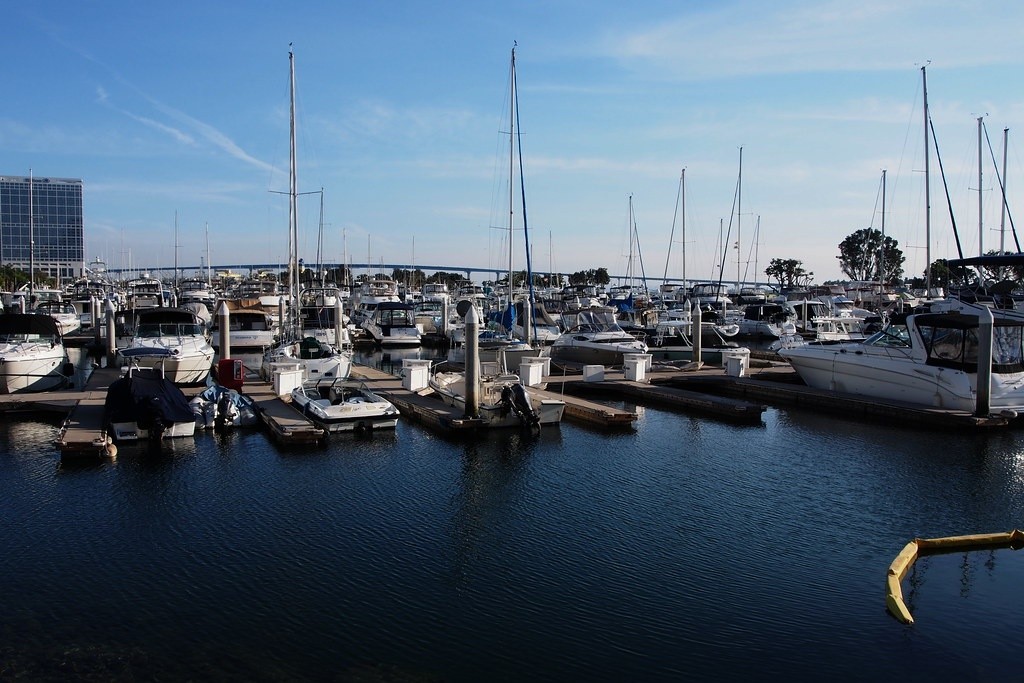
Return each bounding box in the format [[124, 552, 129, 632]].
[[0, 41, 1024, 459], [784, 300, 1023, 413]]
